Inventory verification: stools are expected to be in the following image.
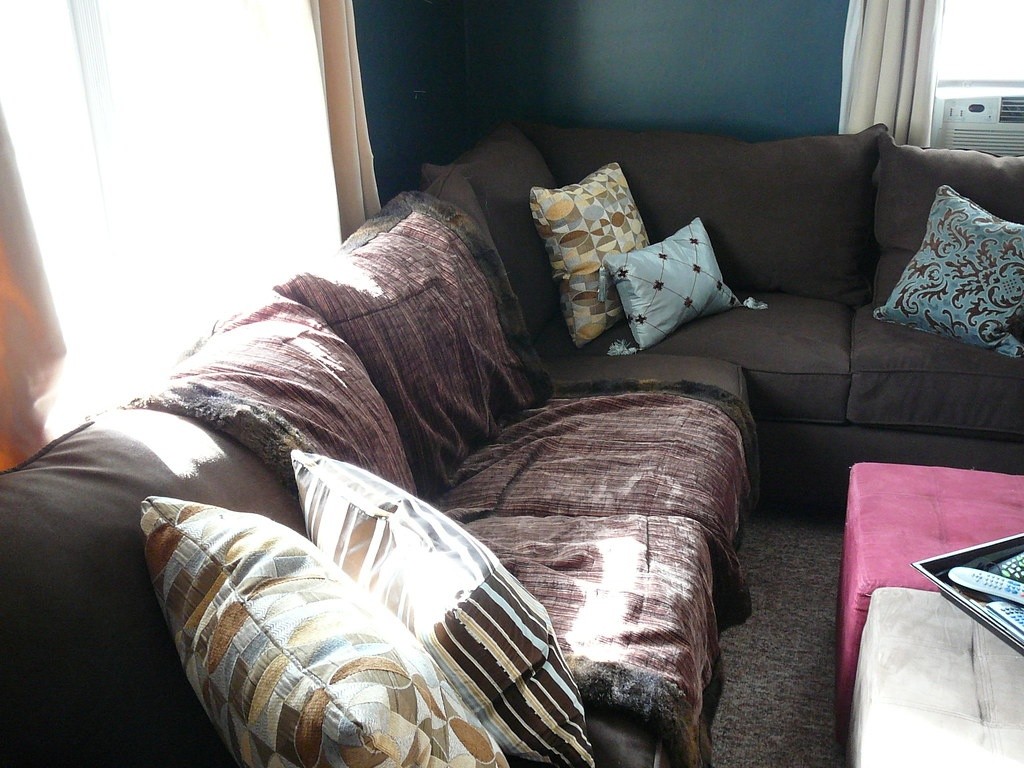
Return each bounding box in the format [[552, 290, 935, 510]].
[[846, 588, 1024, 768], [835, 461, 1024, 750]]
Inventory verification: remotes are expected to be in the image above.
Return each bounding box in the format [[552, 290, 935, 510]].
[[948, 550, 1024, 636]]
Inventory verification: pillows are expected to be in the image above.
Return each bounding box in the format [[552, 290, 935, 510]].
[[528, 160, 650, 349], [597, 217, 769, 357], [136, 493, 510, 768], [871, 184, 1024, 358], [288, 448, 596, 768]]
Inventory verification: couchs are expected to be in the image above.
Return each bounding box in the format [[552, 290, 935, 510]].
[[0, 118, 1024, 768]]
